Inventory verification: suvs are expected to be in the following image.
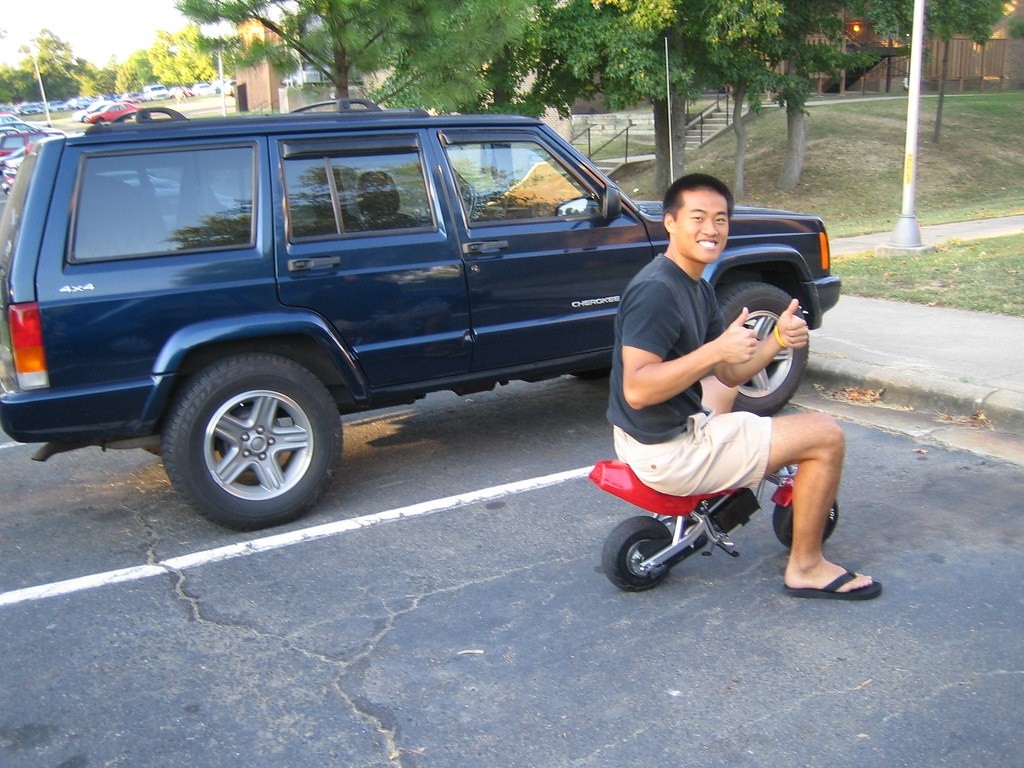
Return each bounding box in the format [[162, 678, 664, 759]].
[[0, 97, 842, 532]]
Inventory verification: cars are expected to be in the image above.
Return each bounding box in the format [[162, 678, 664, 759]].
[[169, 85, 195, 100], [0, 92, 147, 116], [87, 103, 145, 124], [211, 78, 239, 97], [71, 101, 117, 123], [0, 114, 86, 197], [191, 83, 216, 96], [91, 171, 181, 196]]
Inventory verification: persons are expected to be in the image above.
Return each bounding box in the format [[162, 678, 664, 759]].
[[604, 173, 883, 601]]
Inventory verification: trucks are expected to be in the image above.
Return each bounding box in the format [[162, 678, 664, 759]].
[[142, 85, 172, 101]]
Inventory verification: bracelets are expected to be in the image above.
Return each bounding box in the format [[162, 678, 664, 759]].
[[773, 324, 788, 347]]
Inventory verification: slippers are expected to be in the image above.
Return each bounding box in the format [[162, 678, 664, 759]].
[[783, 563, 882, 600]]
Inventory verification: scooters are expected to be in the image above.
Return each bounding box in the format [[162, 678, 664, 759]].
[[589, 456, 838, 592]]
